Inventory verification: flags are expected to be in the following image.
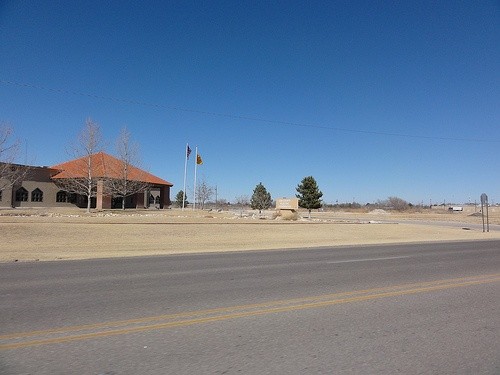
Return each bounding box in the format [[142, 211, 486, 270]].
[[187, 145, 193, 160], [196, 153, 204, 168]]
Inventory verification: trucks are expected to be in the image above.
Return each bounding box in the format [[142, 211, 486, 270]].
[[447, 206, 463, 212]]
